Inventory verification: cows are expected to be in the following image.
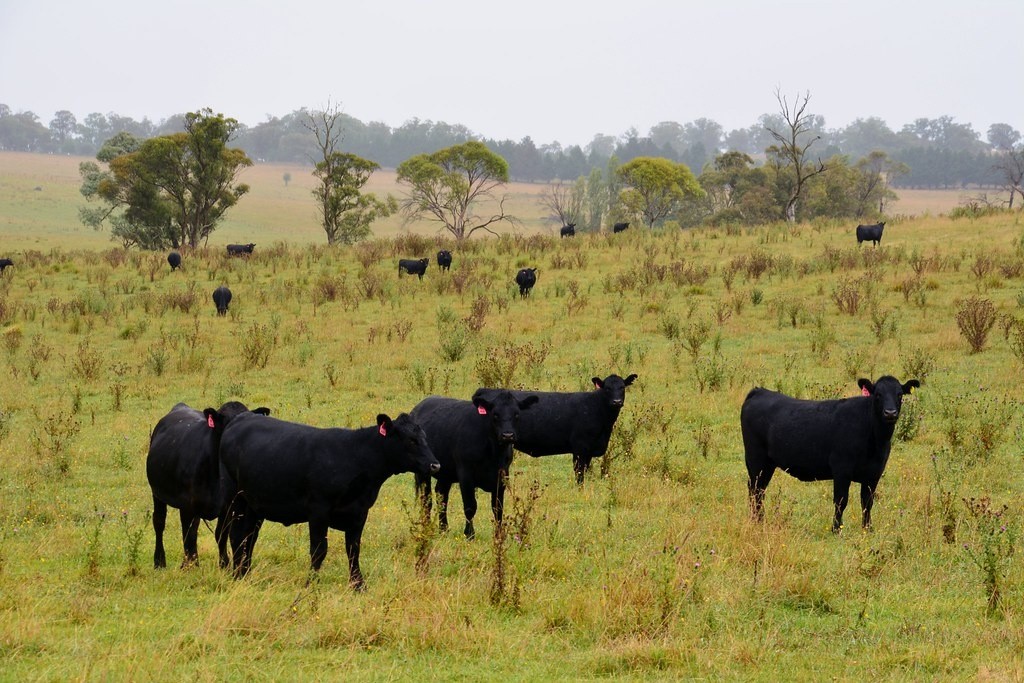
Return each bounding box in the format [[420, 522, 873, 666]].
[[854, 219, 887, 251], [144, 372, 640, 593], [166, 242, 258, 318], [396, 247, 453, 282], [0, 256, 15, 275], [557, 221, 631, 241], [738, 373, 921, 540], [514, 266, 539, 301]]
